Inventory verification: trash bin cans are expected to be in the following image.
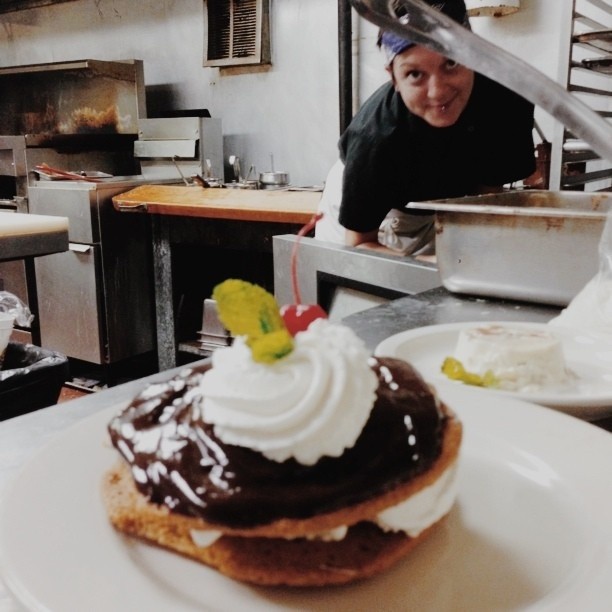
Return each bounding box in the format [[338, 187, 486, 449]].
[[0, 339, 69, 421]]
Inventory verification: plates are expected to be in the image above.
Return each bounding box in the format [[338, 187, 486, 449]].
[[373, 321, 612, 424], [1, 381, 612, 610]]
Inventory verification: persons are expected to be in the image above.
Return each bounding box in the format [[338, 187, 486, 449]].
[[314, 0, 537, 263]]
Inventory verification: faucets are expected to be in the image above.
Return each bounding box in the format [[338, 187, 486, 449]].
[[228, 154, 242, 182]]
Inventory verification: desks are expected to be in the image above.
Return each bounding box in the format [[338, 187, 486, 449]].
[[0, 212, 69, 346], [0, 286, 611, 612]]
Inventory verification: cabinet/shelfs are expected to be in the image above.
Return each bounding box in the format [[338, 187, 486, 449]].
[[111, 184, 323, 373]]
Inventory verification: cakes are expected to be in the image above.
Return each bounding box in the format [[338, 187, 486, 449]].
[[99, 277, 464, 587], [452, 321, 575, 391]]
